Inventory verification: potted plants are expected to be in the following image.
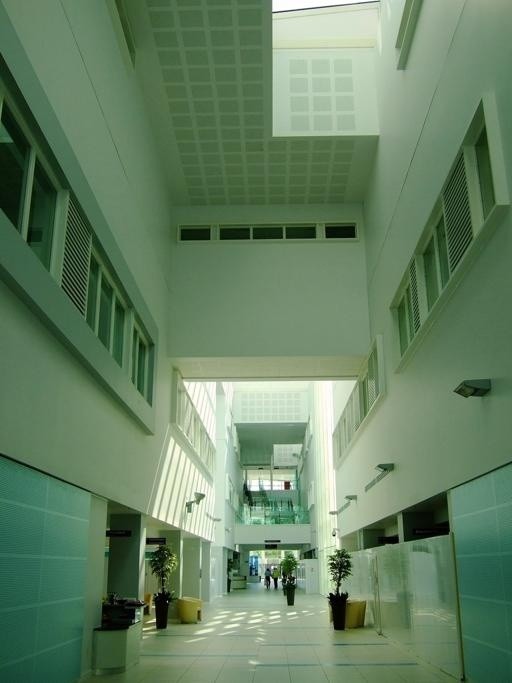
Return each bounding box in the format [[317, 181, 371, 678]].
[[327, 549, 353, 633], [280, 555, 298, 606], [148, 544, 180, 629], [226, 557, 234, 593]]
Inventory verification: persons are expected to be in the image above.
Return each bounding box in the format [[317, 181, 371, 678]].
[[272, 566, 280, 588], [265, 568, 272, 588]]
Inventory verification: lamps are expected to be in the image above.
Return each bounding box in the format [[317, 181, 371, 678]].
[[454, 377, 491, 400], [375, 463, 394, 473], [186, 492, 206, 513]]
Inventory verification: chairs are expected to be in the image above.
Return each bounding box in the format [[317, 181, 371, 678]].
[[179, 596, 203, 624], [331, 596, 367, 630]]
